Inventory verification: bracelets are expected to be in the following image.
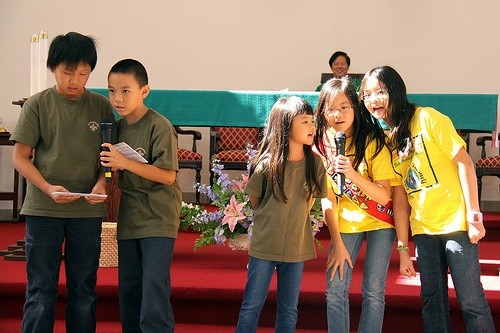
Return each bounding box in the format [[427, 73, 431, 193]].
[[396, 245, 412, 253]]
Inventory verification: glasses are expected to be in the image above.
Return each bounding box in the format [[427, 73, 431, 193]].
[[361, 90, 388, 102], [323, 104, 355, 115]]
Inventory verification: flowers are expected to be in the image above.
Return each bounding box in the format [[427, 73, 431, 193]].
[[178, 143, 326, 251]]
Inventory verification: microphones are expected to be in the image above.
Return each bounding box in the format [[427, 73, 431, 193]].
[[334, 131, 347, 196], [101, 117, 113, 183]]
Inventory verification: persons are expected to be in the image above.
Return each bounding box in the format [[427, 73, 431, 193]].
[[314, 77, 395, 332], [314, 50, 364, 92], [235, 96, 328, 332], [359, 66, 499, 332], [96, 59, 183, 333], [12, 31, 118, 333]]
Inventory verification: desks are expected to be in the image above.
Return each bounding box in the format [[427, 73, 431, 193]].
[[13, 90, 500, 267]]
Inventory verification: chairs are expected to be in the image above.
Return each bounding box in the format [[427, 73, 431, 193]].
[[0, 132, 19, 223], [475, 135, 500, 206], [175, 125, 202, 207], [209, 126, 264, 204]]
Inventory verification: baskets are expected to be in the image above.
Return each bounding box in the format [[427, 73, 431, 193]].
[[98, 221, 119, 268]]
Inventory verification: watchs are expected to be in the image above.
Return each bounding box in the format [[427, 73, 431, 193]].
[[465, 211, 483, 225]]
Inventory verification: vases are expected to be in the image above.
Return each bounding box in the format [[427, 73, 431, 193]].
[[230, 234, 252, 251]]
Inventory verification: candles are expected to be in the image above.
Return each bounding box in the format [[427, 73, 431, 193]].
[[30, 34, 39, 96], [38, 30, 49, 92]]
